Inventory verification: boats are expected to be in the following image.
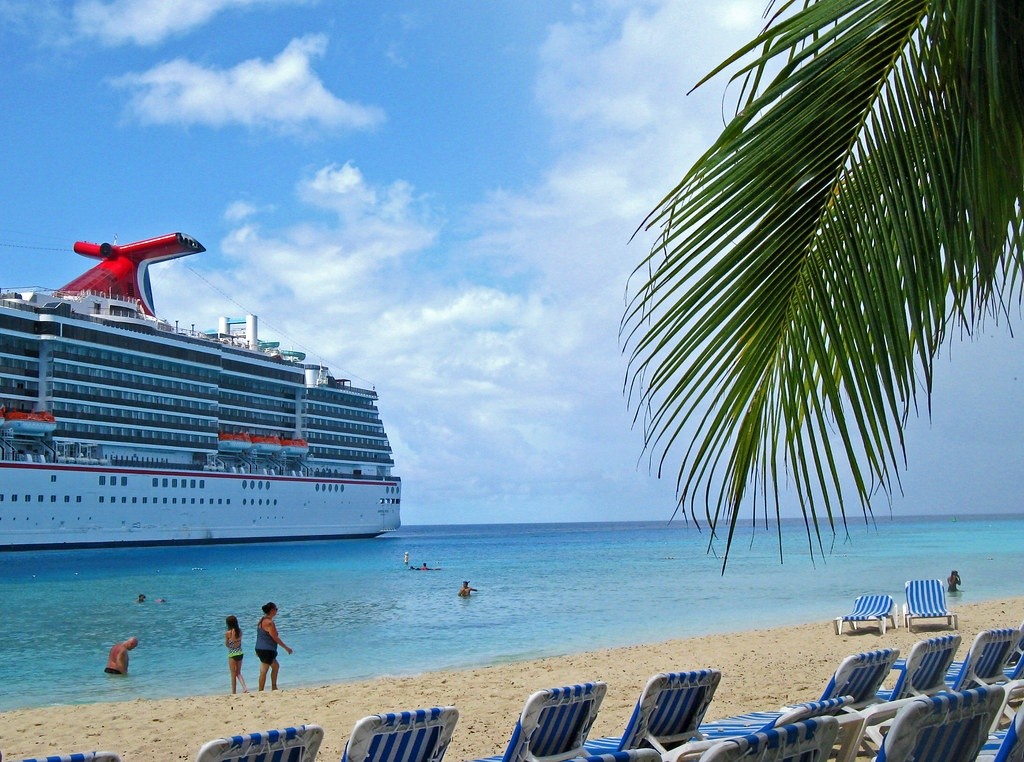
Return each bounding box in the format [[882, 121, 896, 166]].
[[277, 438, 309, 458], [250, 436, 282, 457], [218, 432, 252, 454], [4, 410, 56, 437]]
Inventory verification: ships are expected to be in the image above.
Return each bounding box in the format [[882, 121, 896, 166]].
[[1, 231, 404, 548]]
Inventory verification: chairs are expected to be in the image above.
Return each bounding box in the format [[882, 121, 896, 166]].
[[0, 621, 1024, 762], [833, 595, 898, 634], [902, 580, 958, 633]]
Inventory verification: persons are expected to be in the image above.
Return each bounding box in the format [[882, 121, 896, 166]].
[[137, 595, 145, 603], [225, 616, 248, 695], [104, 637, 137, 675], [458, 581, 477, 596], [255, 603, 293, 691], [947, 571, 961, 591], [404, 552, 408, 564], [422, 563, 428, 570]]
